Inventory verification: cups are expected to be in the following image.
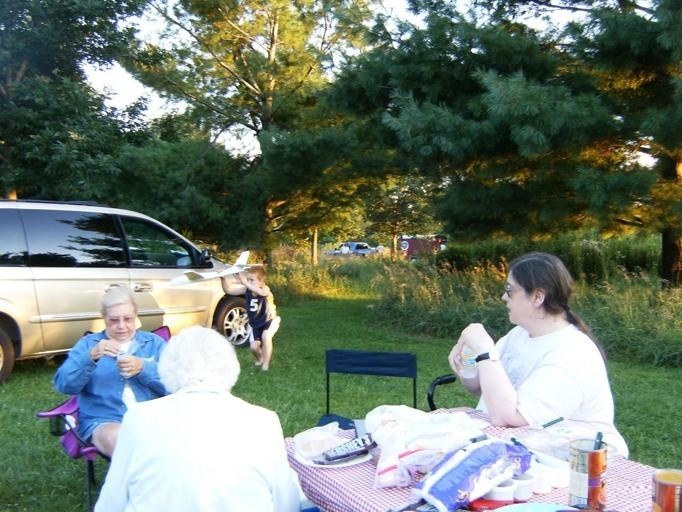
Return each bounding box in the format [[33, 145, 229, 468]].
[[512, 474, 534, 503], [566, 438, 608, 511], [482, 479, 515, 501], [528, 465, 553, 494], [650, 467, 682, 512]]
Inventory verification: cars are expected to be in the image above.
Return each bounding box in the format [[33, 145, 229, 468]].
[[323, 231, 448, 261]]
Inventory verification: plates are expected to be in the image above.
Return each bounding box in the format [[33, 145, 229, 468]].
[[295, 448, 372, 468], [493, 502, 576, 512]]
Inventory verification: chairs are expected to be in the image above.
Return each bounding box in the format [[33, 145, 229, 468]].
[[314, 348, 419, 429], [427, 373, 459, 411], [36, 324, 174, 512]]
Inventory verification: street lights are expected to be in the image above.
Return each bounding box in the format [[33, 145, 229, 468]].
[[389, 184, 414, 257]]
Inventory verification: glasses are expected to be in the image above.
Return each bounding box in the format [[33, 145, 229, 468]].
[[504, 283, 521, 297]]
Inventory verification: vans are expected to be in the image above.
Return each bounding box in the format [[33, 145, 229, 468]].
[[0, 197, 253, 384]]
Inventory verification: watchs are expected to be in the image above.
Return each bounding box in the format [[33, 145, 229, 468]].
[[475, 346, 500, 364]]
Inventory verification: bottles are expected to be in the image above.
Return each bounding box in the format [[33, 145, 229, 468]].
[[459, 339, 477, 379]]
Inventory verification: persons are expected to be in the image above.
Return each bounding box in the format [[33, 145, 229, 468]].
[[92, 325, 305, 512], [51, 283, 172, 459], [219, 262, 281, 371], [447, 252, 628, 461]]
